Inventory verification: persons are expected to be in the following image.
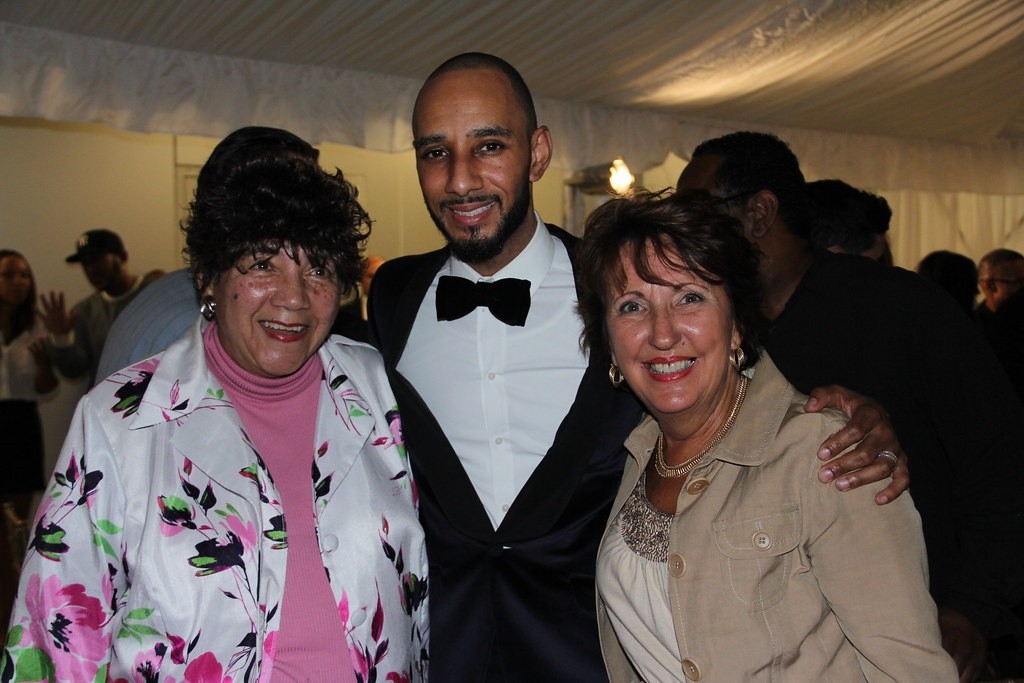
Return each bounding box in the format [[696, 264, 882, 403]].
[[575, 188, 959, 683], [0, 125, 429, 683], [677, 130, 1024, 683], [325, 52, 910, 683]]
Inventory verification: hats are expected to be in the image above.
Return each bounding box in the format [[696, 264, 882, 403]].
[[64, 229, 128, 262]]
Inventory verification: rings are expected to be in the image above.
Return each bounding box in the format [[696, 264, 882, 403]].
[[879, 450, 898, 463]]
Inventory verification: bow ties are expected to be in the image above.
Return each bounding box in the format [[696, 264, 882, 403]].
[[435, 274, 531, 327]]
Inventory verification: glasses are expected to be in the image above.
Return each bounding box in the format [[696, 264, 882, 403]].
[[697, 194, 739, 215], [991, 277, 1023, 286]]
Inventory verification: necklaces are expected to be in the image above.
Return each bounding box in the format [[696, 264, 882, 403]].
[[653, 376, 748, 477]]
